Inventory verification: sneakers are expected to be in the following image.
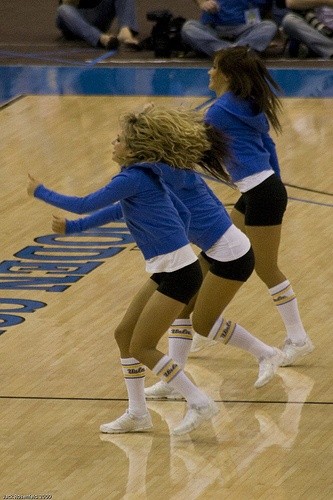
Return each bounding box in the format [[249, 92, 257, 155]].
[[171, 397, 221, 435], [189, 332, 222, 353], [99, 408, 154, 433], [254, 347, 285, 389], [143, 380, 185, 400], [278, 336, 315, 367]]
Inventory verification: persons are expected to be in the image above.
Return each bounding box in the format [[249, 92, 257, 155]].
[[188, 47, 315, 366], [26, 103, 220, 436], [143, 120, 288, 400], [55, 0, 333, 61]]
